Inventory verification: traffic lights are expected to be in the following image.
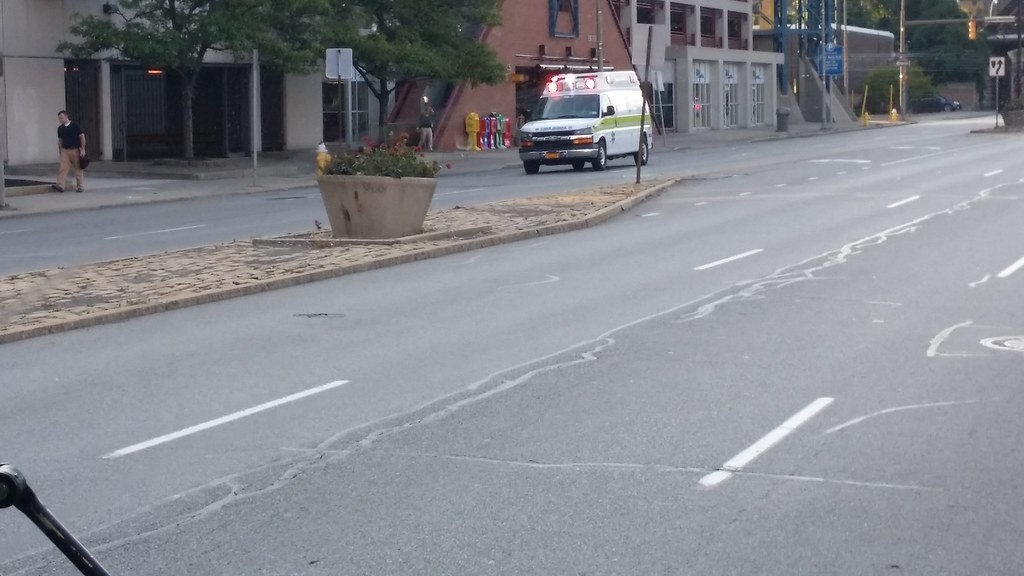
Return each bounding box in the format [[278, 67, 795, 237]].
[[968, 20, 978, 40]]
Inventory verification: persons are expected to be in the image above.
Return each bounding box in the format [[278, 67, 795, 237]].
[[51, 110, 90, 192], [417, 108, 434, 152]]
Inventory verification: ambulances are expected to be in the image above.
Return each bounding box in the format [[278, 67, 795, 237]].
[[518, 70, 654, 175]]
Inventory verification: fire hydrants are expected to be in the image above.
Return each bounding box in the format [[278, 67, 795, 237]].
[[891, 108, 899, 121]]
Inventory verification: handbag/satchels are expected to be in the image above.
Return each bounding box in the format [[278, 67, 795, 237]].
[[79, 154, 90, 170]]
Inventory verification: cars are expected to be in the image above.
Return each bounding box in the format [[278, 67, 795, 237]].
[[910, 95, 962, 114]]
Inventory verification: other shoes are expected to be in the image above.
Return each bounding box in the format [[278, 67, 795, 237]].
[[76, 188, 84, 192], [52, 184, 65, 193]]
